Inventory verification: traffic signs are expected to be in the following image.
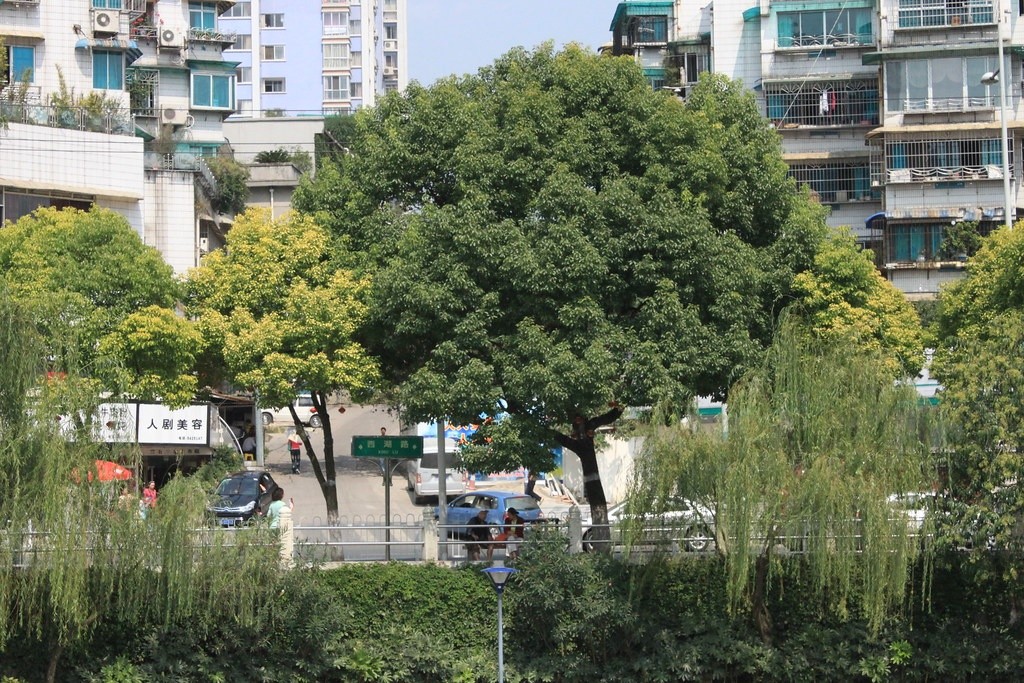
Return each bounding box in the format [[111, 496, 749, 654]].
[[351, 435, 423, 561]]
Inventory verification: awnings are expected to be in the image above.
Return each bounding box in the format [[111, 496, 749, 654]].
[[0, 30, 45, 40], [76, 38, 143, 68], [865, 213, 885, 229], [218, 0, 236, 17]]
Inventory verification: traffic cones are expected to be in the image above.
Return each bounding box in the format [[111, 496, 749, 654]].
[[468, 471, 477, 490]]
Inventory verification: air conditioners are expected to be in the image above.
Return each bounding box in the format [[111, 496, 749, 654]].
[[92, 10, 120, 33], [383, 67, 394, 73], [160, 103, 188, 124], [159, 26, 185, 47], [384, 42, 395, 50]]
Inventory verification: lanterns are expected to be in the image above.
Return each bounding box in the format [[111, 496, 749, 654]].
[[339, 407, 345, 413], [310, 407, 316, 413]]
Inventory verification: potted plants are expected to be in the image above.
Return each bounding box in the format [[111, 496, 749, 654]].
[[191, 30, 237, 41], [130, 104, 142, 117]]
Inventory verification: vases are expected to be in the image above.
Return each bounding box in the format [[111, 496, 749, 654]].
[[140, 29, 147, 36]]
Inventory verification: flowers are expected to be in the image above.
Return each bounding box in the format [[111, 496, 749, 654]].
[[131, 11, 164, 36]]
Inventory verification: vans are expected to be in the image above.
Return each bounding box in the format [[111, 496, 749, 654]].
[[406, 438, 466, 504], [262, 394, 322, 428]]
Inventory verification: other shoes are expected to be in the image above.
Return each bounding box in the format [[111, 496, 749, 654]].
[[537, 499, 544, 506], [296, 469, 300, 474]]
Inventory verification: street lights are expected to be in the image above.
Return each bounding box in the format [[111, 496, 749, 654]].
[[480, 560, 517, 683], [981, 0, 1012, 232]]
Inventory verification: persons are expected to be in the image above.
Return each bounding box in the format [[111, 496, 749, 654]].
[[242, 434, 256, 459], [117, 480, 159, 528], [379, 427, 387, 476], [526, 473, 542, 504], [465, 512, 494, 561], [249, 482, 266, 493], [266, 488, 294, 542], [504, 507, 525, 562], [245, 419, 253, 436], [288, 431, 302, 474]]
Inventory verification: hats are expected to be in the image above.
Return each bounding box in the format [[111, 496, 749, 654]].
[[480, 508, 489, 512], [507, 507, 519, 514]]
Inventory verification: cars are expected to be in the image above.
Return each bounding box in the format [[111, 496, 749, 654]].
[[856, 490, 997, 548], [433, 491, 545, 547], [581, 494, 716, 552], [204, 470, 279, 528]]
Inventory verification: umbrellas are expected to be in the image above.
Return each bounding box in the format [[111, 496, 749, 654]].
[[70, 459, 133, 483]]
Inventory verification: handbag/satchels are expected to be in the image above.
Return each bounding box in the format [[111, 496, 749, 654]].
[[288, 444, 291, 450]]
